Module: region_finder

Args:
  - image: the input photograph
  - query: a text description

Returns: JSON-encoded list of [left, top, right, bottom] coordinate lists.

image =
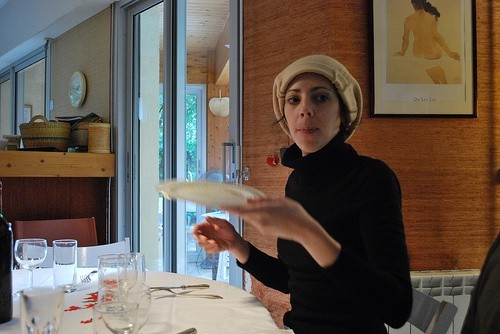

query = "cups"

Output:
[[53, 239, 77, 293], [93, 301, 139, 334], [20, 286, 64, 334], [88, 123, 110, 153]]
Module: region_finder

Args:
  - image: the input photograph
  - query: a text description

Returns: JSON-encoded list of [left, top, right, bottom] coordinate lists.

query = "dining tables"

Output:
[[0, 267, 294, 333]]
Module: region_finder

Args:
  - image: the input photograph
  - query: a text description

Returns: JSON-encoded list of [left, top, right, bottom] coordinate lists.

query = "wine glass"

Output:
[[14, 239, 47, 296], [98, 252, 150, 334]]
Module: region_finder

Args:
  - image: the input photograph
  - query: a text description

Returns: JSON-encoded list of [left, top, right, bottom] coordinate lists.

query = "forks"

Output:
[[80, 271, 98, 282], [152, 288, 223, 300]]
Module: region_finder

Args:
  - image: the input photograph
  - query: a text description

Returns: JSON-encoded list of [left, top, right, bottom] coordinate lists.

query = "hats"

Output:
[[272, 55, 363, 144]]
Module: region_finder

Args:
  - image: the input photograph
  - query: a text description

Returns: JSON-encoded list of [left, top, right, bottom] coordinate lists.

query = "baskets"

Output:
[[19, 115, 71, 152]]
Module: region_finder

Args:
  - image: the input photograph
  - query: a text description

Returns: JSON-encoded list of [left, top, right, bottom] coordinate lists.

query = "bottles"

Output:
[[0, 182, 13, 324]]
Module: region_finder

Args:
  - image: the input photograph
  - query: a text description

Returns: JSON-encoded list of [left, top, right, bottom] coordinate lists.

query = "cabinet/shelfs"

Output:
[[1, 148, 116, 247]]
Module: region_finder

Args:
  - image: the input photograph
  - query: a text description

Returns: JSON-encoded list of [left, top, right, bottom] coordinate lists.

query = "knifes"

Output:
[[149, 284, 209, 289]]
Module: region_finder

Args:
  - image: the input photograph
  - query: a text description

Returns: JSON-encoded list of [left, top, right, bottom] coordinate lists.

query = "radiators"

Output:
[[381, 268, 484, 334]]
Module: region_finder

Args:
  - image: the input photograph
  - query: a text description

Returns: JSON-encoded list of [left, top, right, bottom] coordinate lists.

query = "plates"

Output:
[[158, 181, 267, 207]]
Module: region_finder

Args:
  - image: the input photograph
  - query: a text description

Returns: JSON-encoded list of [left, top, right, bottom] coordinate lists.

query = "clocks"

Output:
[[68, 72, 86, 108]]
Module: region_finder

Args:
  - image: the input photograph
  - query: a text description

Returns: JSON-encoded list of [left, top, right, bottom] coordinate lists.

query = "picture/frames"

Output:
[[366, 0, 479, 119]]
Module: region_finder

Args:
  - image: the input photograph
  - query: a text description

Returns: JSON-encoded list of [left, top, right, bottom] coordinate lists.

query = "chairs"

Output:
[[7, 216, 99, 268], [397, 288, 458, 333], [21, 237, 131, 269]]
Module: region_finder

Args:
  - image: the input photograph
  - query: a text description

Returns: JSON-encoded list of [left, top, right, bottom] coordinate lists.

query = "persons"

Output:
[[191, 54, 413, 334]]
